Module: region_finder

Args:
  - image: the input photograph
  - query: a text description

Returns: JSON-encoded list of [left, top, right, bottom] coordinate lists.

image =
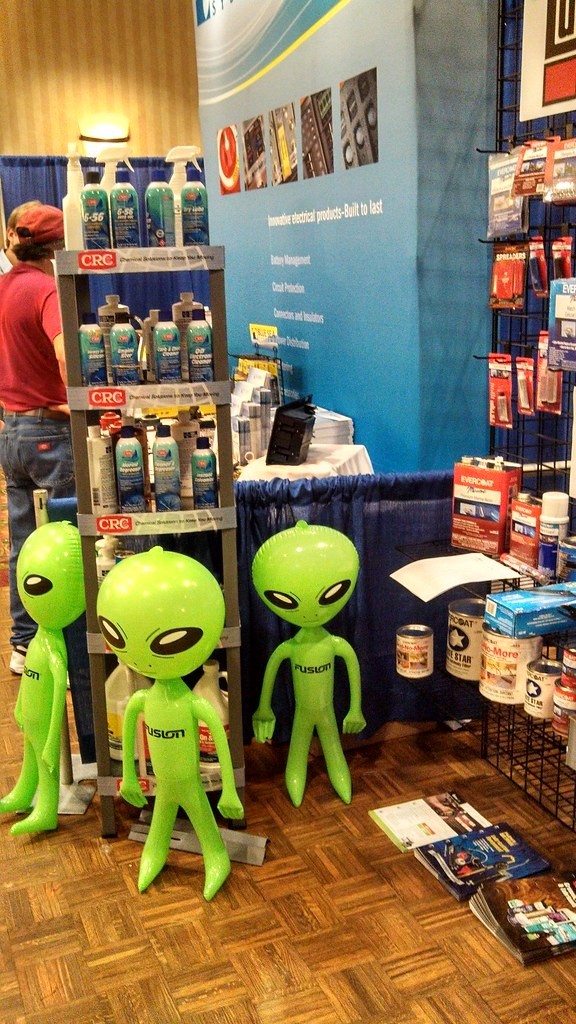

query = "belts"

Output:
[[3, 407, 68, 422]]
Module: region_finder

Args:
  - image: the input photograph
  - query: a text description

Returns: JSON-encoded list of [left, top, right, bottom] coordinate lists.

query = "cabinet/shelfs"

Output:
[[54, 247, 246, 837]]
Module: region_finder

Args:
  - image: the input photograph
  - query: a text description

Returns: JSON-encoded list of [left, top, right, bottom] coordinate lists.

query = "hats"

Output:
[[15, 205, 64, 245]]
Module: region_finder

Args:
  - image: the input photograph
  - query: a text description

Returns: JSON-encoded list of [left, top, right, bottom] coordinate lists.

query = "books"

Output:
[[212, 365, 356, 467], [368, 790, 576, 967]]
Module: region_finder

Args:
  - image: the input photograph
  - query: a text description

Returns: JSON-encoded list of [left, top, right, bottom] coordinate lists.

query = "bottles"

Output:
[[103, 656, 229, 781]]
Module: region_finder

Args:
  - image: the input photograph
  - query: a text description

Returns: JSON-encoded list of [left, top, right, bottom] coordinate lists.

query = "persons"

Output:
[[0, 200, 85, 686]]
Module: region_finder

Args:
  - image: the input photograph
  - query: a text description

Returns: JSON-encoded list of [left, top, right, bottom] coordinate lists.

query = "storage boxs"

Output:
[[484, 581, 576, 640], [448, 461, 520, 556]]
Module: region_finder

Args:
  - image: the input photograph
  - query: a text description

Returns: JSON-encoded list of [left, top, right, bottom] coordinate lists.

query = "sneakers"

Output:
[[10, 642, 27, 674]]
